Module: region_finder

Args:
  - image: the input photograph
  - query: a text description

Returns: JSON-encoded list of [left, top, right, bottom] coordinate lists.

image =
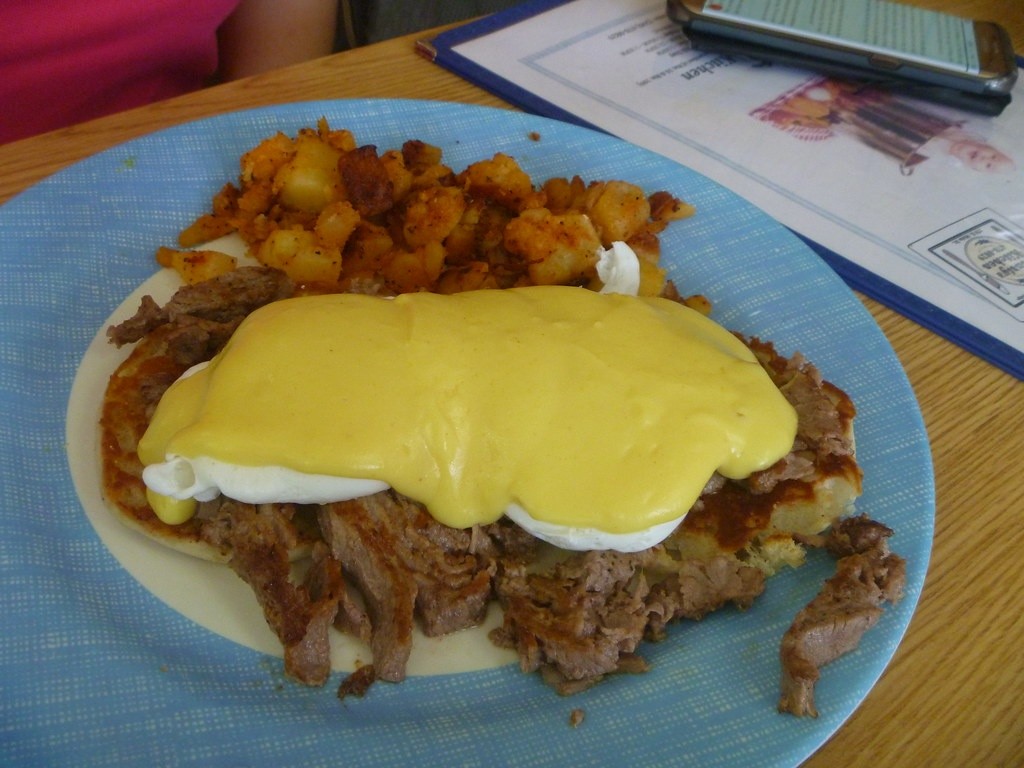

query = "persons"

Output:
[[0, 0, 340, 147]]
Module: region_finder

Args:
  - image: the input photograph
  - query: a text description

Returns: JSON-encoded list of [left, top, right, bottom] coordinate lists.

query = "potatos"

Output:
[[152, 113, 698, 293]]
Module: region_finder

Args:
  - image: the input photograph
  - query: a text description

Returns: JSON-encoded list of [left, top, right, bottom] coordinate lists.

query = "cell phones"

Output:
[[663, 0, 1018, 100]]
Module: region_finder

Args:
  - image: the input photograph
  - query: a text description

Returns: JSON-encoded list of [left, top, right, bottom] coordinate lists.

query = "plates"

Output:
[[0, 98, 935, 768]]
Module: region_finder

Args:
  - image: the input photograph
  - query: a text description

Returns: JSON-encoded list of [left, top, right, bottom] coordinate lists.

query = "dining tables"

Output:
[[0, 0, 1024, 768]]
